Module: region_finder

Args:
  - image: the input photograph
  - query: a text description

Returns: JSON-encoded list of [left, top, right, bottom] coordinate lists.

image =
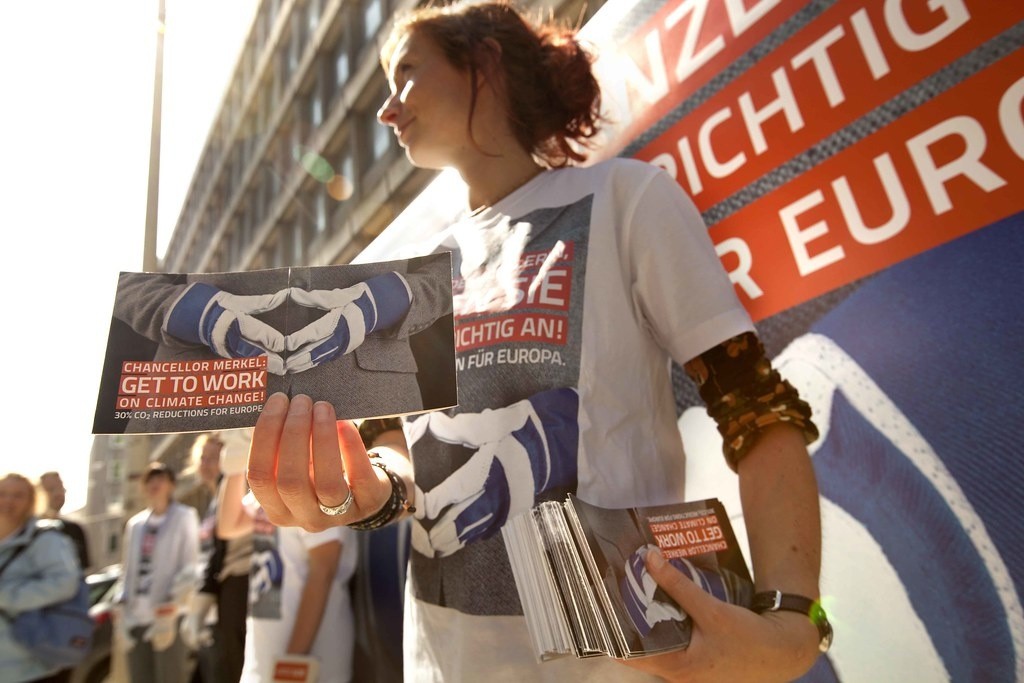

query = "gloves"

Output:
[[422, 387, 581, 559], [162, 282, 291, 377], [619, 544, 730, 639], [286, 271, 413, 376], [399, 412, 437, 560]]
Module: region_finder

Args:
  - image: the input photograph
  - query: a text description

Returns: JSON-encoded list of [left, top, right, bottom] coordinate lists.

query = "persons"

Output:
[[248, 0, 822, 683], [0, 435, 360, 683], [113, 252, 453, 435], [579, 497, 753, 652]]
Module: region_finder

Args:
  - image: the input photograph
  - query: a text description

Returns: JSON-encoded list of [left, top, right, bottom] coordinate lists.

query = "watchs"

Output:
[[754, 590, 834, 653]]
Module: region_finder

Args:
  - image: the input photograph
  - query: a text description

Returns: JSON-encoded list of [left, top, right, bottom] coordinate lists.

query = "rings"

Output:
[[318, 484, 355, 516]]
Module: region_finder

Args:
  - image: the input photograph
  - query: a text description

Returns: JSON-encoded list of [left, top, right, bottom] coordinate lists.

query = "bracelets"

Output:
[[346, 452, 416, 533]]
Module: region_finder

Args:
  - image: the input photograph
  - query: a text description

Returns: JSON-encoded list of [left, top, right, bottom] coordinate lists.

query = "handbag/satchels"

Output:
[[1, 527, 93, 671]]
